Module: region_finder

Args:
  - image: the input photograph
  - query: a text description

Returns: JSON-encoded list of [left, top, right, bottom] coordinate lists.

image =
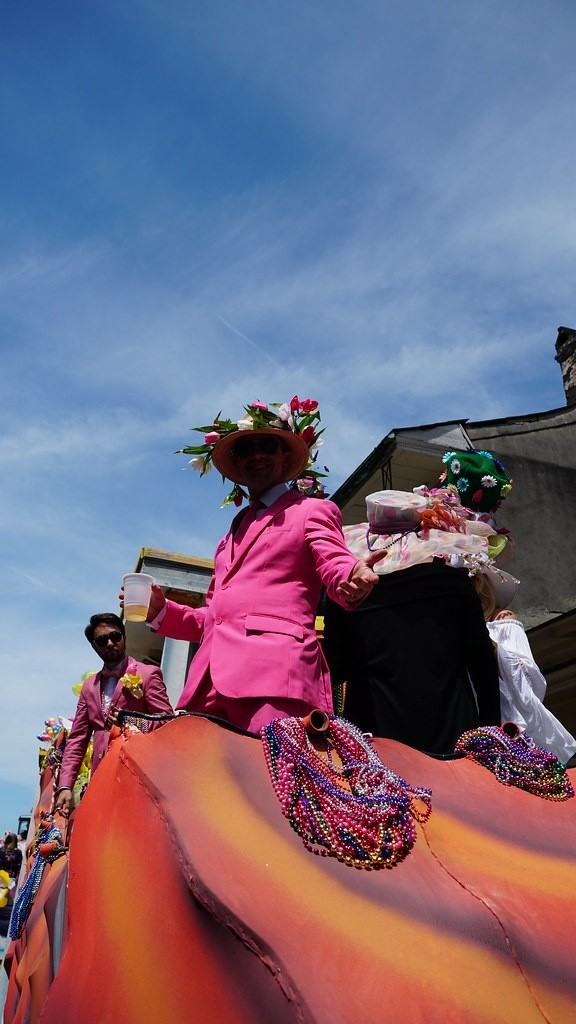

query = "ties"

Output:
[[232, 499, 263, 561]]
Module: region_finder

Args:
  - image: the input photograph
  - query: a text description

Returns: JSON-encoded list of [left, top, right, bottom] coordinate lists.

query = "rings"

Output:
[[56, 806, 61, 810]]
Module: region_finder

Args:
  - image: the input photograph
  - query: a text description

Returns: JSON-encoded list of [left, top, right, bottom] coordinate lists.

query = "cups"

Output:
[[123, 573, 155, 622]]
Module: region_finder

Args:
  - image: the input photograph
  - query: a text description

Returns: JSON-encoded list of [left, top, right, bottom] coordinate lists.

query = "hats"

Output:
[[342, 489, 488, 575], [212, 411, 309, 488]]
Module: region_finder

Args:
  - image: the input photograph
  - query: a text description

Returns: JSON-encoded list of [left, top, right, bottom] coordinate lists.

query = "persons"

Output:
[[471, 570, 576, 768], [0, 833, 23, 878], [54, 611, 172, 819], [119, 422, 385, 736]]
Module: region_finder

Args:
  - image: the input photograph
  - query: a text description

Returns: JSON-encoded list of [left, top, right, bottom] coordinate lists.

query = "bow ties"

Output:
[[101, 668, 122, 678]]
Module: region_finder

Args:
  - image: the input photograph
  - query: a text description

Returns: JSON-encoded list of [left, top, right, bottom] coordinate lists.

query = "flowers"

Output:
[[173, 394, 331, 510], [438, 448, 514, 513]]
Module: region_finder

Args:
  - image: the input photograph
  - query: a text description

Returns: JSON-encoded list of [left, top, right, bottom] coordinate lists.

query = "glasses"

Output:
[[234, 436, 285, 457], [93, 631, 122, 647]]
